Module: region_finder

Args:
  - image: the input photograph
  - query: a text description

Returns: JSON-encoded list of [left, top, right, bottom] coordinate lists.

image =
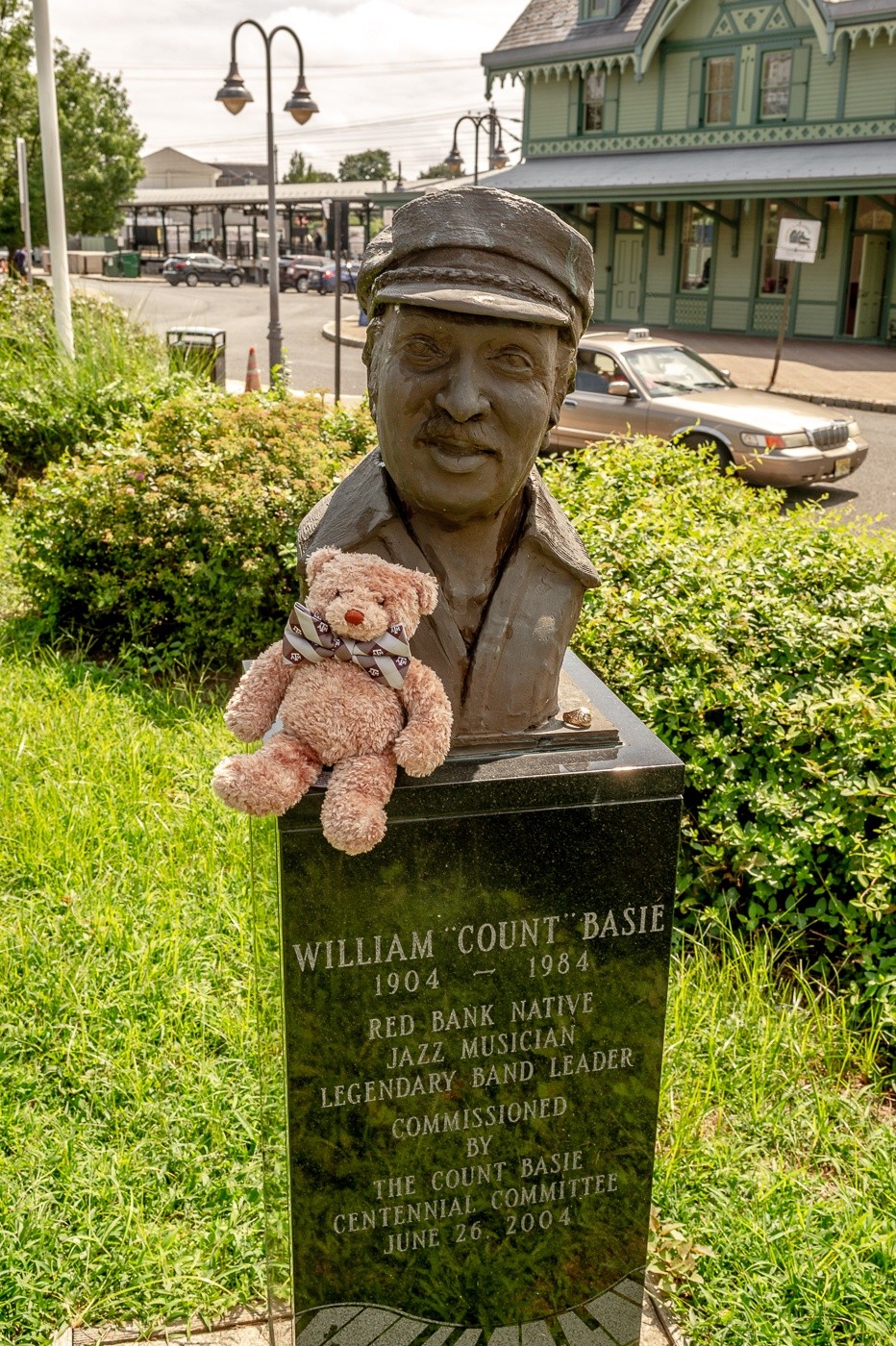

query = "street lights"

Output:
[[212, 18, 320, 390], [444, 115, 512, 187]]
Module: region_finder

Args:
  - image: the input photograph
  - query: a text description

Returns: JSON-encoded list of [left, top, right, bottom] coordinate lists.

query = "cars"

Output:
[[539, 328, 869, 490]]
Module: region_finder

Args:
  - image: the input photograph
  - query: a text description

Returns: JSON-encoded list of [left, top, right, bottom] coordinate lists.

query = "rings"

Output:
[[563, 707, 592, 728]]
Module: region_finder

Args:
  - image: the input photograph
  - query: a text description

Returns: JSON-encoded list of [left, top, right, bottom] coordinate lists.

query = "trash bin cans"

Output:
[[163, 327, 225, 386], [120, 252, 140, 279], [102, 255, 119, 277]]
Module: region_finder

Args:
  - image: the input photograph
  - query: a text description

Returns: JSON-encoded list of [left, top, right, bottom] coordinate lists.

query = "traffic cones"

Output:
[[245, 347, 263, 391]]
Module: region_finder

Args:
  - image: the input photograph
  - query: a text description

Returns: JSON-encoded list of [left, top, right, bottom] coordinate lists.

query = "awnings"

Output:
[[461, 139, 896, 204]]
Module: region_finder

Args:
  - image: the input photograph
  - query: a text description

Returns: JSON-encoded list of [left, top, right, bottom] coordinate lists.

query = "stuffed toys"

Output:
[[210, 548, 449, 854]]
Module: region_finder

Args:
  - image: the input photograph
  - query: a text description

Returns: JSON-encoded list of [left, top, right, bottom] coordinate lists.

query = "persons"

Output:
[[292, 187, 602, 736], [314, 231, 322, 250]]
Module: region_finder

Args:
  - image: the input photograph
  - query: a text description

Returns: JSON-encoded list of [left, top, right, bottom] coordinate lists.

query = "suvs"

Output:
[[162, 253, 247, 287], [265, 254, 364, 296]]
